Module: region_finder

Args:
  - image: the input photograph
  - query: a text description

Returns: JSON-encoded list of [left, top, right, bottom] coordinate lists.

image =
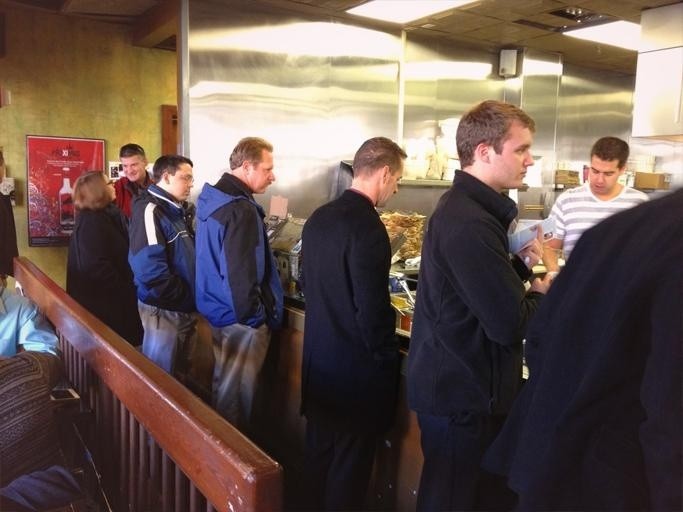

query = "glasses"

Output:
[[170, 171, 194, 184]]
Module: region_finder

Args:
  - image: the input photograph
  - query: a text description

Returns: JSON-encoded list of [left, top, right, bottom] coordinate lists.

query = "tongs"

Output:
[[399, 279, 415, 310]]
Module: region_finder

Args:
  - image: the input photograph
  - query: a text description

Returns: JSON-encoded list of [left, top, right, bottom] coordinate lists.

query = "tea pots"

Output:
[[402, 119, 448, 180]]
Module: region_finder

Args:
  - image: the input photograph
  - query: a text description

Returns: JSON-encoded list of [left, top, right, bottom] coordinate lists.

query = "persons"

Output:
[[112, 143, 152, 221], [66, 170, 144, 348], [1, 349, 61, 489], [506, 178, 683, 512], [539, 136, 651, 283], [128, 154, 198, 377], [0, 272, 60, 353], [298, 137, 408, 511], [195, 136, 284, 450], [406, 99, 553, 512]]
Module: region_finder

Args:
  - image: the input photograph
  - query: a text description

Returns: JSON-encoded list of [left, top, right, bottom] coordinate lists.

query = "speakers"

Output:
[[499, 48, 517, 77]]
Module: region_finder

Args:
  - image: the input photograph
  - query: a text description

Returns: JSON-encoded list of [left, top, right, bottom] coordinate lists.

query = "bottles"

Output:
[[59, 167, 75, 225]]
